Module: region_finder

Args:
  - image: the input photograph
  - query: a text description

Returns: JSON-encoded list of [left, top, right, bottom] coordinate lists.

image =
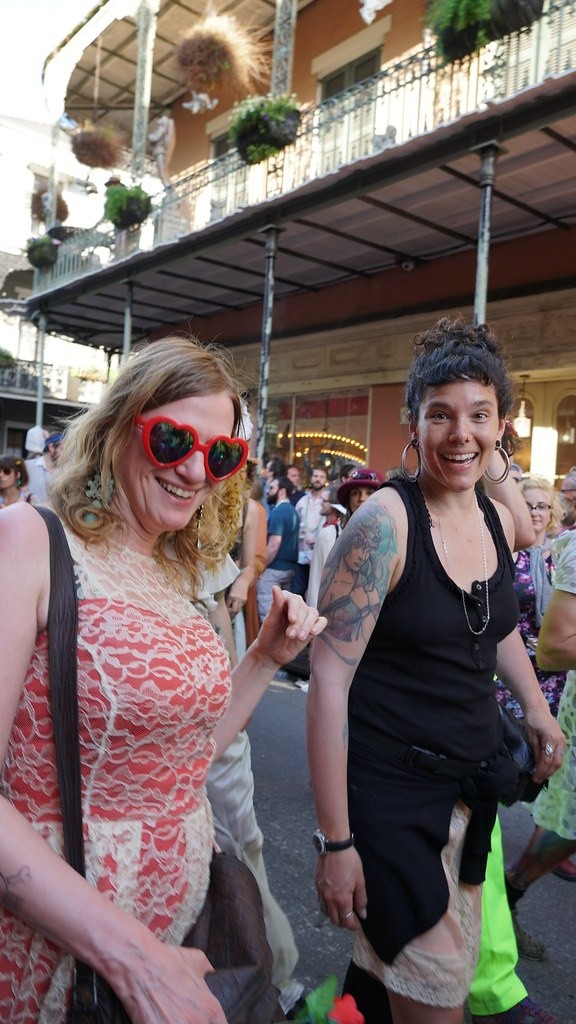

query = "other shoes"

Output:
[[549, 857, 576, 883], [510, 906, 548, 961], [471, 996, 561, 1024]]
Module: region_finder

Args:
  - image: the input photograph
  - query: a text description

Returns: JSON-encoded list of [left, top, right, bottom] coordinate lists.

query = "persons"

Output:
[[182, 91, 219, 115], [78, 175, 98, 196], [95, 173, 120, 228], [0, 432, 576, 1023], [147, 110, 176, 188], [305, 319, 567, 1024], [0, 334, 328, 1023]]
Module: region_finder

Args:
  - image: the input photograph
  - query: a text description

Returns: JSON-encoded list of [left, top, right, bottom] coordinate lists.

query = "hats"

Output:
[[336, 469, 386, 510], [321, 486, 348, 515]]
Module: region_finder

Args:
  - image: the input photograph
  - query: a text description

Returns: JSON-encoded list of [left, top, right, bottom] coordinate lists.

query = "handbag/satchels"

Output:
[[67, 851, 289, 1024]]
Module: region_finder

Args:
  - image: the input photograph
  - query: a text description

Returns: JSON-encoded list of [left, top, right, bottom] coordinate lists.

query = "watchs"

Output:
[[310, 829, 355, 857]]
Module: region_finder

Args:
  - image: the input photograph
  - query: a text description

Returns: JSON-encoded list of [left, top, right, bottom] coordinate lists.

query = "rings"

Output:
[[542, 740, 553, 756], [341, 911, 352, 921]]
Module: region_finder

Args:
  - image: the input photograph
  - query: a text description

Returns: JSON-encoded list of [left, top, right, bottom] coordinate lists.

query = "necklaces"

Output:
[[424, 487, 491, 635]]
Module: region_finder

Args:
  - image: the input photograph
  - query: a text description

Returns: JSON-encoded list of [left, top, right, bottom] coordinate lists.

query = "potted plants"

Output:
[[106, 186, 149, 224], [228, 97, 304, 163], [429, 0, 543, 63], [22, 237, 58, 270]]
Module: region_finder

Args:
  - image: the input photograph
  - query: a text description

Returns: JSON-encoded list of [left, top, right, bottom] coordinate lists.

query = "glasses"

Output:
[[350, 471, 383, 485], [526, 501, 551, 512], [0, 468, 17, 476], [132, 414, 249, 481]]
